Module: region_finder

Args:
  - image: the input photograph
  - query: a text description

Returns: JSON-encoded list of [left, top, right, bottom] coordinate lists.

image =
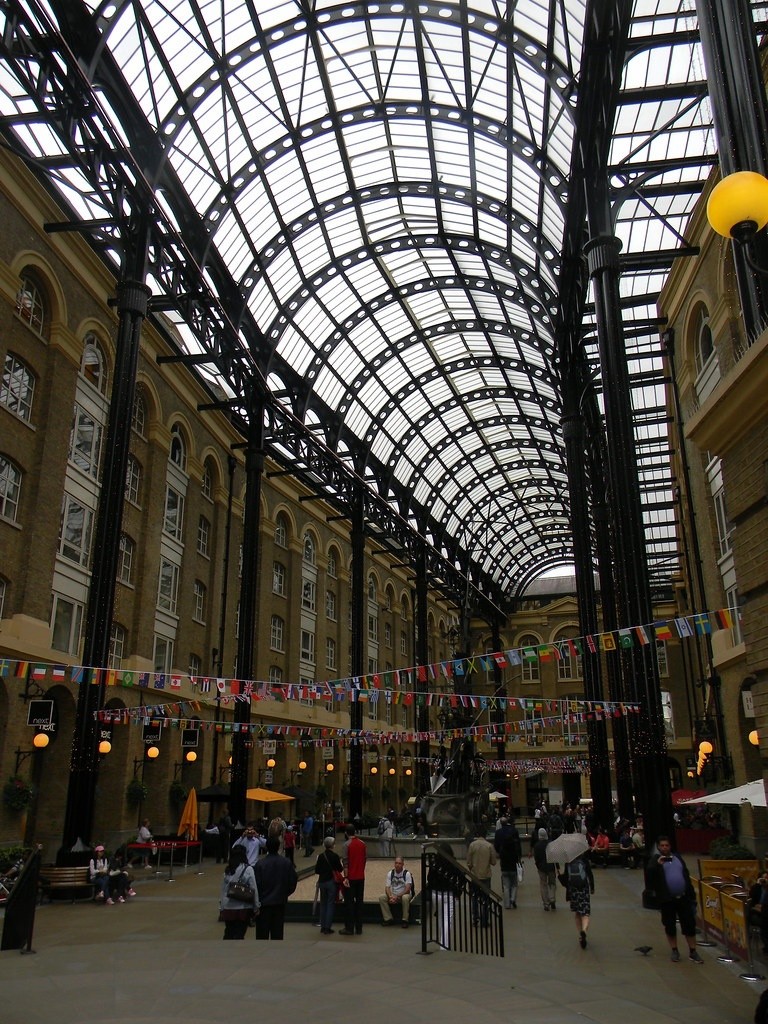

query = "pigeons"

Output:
[[633, 946, 654, 956]]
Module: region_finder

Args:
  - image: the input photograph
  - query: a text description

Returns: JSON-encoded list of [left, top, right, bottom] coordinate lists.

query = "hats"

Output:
[[538, 828, 548, 840], [634, 826, 644, 830], [542, 802, 545, 804], [324, 836, 335, 848], [288, 825, 293, 830], [416, 808, 422, 813], [96, 846, 105, 851]]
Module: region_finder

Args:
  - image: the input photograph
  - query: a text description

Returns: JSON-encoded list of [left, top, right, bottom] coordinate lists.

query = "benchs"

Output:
[[608, 842, 632, 863], [38, 866, 135, 906]]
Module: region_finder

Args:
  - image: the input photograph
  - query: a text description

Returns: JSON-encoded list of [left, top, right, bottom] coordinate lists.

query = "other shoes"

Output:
[[145, 865, 152, 869], [671, 950, 680, 962], [107, 898, 115, 904], [544, 907, 550, 911], [579, 931, 587, 950], [551, 901, 556, 909], [510, 900, 517, 909], [321, 919, 408, 936], [412, 834, 418, 840], [127, 888, 137, 896], [127, 863, 132, 868], [118, 896, 126, 903], [689, 950, 704, 963], [624, 867, 630, 870], [425, 835, 428, 840], [98, 890, 104, 897]]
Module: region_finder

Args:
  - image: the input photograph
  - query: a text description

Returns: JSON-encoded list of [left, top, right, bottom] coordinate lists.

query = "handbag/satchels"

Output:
[[152, 843, 157, 855], [332, 870, 344, 883], [642, 889, 672, 910], [558, 873, 565, 886], [226, 864, 255, 904], [516, 862, 523, 882], [135, 833, 147, 844]]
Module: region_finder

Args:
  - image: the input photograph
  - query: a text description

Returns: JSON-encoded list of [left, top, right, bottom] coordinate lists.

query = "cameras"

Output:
[[663, 856, 672, 862]]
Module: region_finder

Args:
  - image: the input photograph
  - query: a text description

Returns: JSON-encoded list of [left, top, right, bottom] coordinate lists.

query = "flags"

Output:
[[0, 603, 746, 779]]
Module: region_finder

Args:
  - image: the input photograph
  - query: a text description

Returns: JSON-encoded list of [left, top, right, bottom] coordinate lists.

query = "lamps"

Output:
[[748, 728, 759, 753], [87, 740, 112, 775], [400, 769, 412, 783], [705, 170, 768, 275], [173, 750, 199, 779], [365, 767, 379, 785], [686, 740, 735, 783], [291, 761, 307, 782], [14, 732, 48, 776], [259, 758, 276, 782], [219, 757, 232, 779], [318, 763, 339, 780], [383, 767, 396, 783], [133, 746, 160, 778]]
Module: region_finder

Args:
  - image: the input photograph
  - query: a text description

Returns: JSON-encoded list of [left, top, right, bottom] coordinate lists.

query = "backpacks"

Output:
[[86, 857, 105, 884], [391, 869, 415, 901], [377, 820, 388, 835], [564, 856, 587, 887]]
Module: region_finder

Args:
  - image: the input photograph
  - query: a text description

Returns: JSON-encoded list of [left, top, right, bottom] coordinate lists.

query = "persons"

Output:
[[3, 791, 768, 964]]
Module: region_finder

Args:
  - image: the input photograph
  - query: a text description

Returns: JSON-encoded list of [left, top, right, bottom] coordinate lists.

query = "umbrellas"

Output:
[[682, 779, 768, 808], [178, 787, 198, 867], [246, 788, 294, 802]]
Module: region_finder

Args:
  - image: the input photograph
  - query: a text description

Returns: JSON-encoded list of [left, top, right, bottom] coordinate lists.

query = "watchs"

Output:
[[397, 895, 400, 901]]
